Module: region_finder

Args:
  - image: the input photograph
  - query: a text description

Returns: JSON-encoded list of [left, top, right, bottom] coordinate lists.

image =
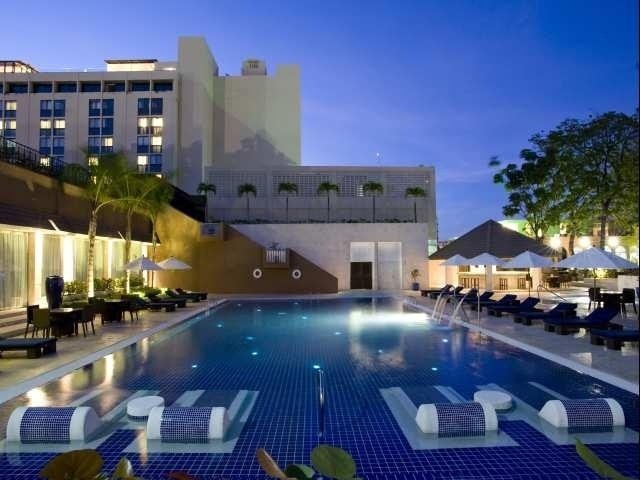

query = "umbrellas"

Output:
[[466, 252, 504, 279], [125, 254, 162, 273], [556, 246, 639, 310], [424, 218, 562, 260], [158, 256, 192, 270], [500, 252, 554, 297], [440, 253, 470, 266]]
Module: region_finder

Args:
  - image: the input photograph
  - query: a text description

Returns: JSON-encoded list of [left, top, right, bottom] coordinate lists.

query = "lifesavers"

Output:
[[253, 269, 262, 279], [292, 268, 302, 279]]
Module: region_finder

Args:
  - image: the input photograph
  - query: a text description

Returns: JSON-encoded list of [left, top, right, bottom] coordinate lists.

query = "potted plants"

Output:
[[411, 269, 420, 291]]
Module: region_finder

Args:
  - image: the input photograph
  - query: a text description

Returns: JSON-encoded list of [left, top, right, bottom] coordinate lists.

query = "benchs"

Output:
[[588, 327, 640, 351], [0, 336, 58, 360]]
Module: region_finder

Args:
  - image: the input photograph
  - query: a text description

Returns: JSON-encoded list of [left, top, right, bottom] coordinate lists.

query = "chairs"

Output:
[[88, 293, 139, 325], [23, 304, 101, 339], [419, 284, 638, 336], [136, 287, 208, 312]]
